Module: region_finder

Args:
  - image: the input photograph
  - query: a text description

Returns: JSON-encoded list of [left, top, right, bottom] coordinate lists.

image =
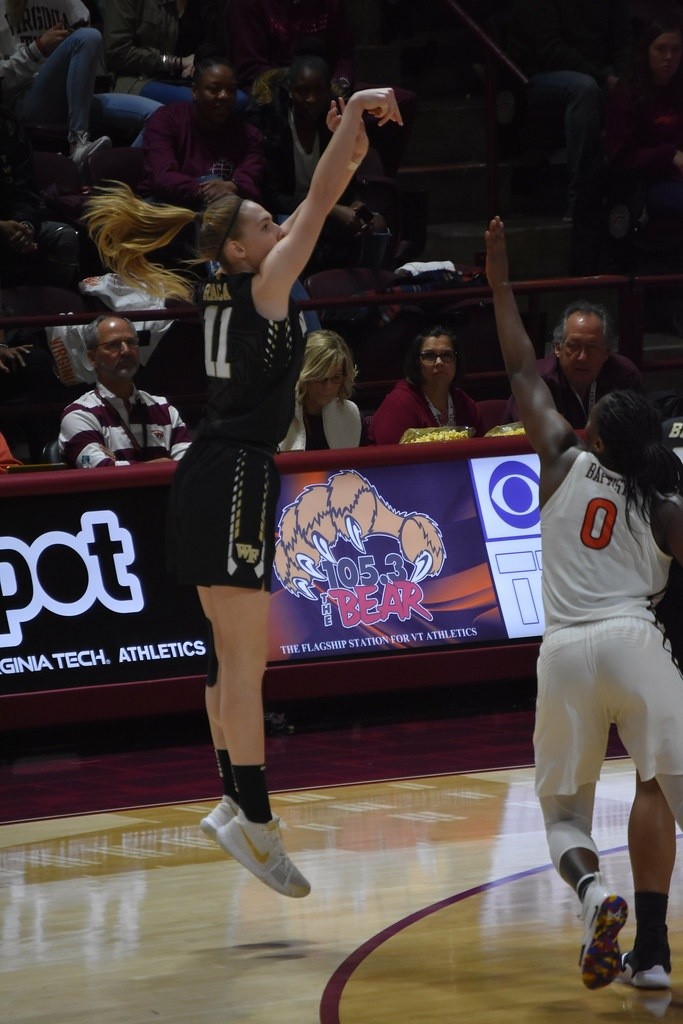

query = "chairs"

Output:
[[0, 0, 682, 433]]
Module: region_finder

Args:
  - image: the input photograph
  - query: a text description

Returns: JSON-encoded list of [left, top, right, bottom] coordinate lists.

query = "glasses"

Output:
[[96, 338, 139, 350], [419, 351, 459, 363], [315, 374, 346, 385]]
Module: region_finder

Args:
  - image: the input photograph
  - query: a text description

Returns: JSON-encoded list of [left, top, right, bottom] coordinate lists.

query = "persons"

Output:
[[485, 215, 683, 990], [0, 0, 683, 444], [81, 83, 403, 898]]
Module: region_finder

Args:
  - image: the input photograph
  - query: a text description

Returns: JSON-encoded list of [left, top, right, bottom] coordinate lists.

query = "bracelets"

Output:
[[0, 344, 8, 348], [350, 159, 359, 172]]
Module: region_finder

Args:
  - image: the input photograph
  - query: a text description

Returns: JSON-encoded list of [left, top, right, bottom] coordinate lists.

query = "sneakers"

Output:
[[200, 794, 240, 841], [615, 950, 671, 990], [578, 872, 628, 990], [215, 809, 310, 897], [71, 135, 112, 177]]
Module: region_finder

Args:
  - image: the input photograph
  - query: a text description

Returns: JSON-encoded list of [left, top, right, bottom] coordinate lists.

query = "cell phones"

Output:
[[356, 205, 374, 223]]
